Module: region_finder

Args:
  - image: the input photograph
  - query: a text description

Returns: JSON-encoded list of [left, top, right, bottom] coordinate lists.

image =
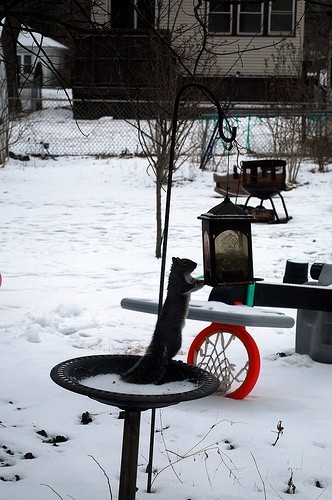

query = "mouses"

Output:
[[119, 256, 204, 384]]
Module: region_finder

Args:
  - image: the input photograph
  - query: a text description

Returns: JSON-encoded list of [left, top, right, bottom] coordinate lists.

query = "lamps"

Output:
[[197, 151, 256, 288]]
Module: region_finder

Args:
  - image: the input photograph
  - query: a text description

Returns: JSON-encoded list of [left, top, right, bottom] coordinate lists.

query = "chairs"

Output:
[[240, 160, 292, 224]]
